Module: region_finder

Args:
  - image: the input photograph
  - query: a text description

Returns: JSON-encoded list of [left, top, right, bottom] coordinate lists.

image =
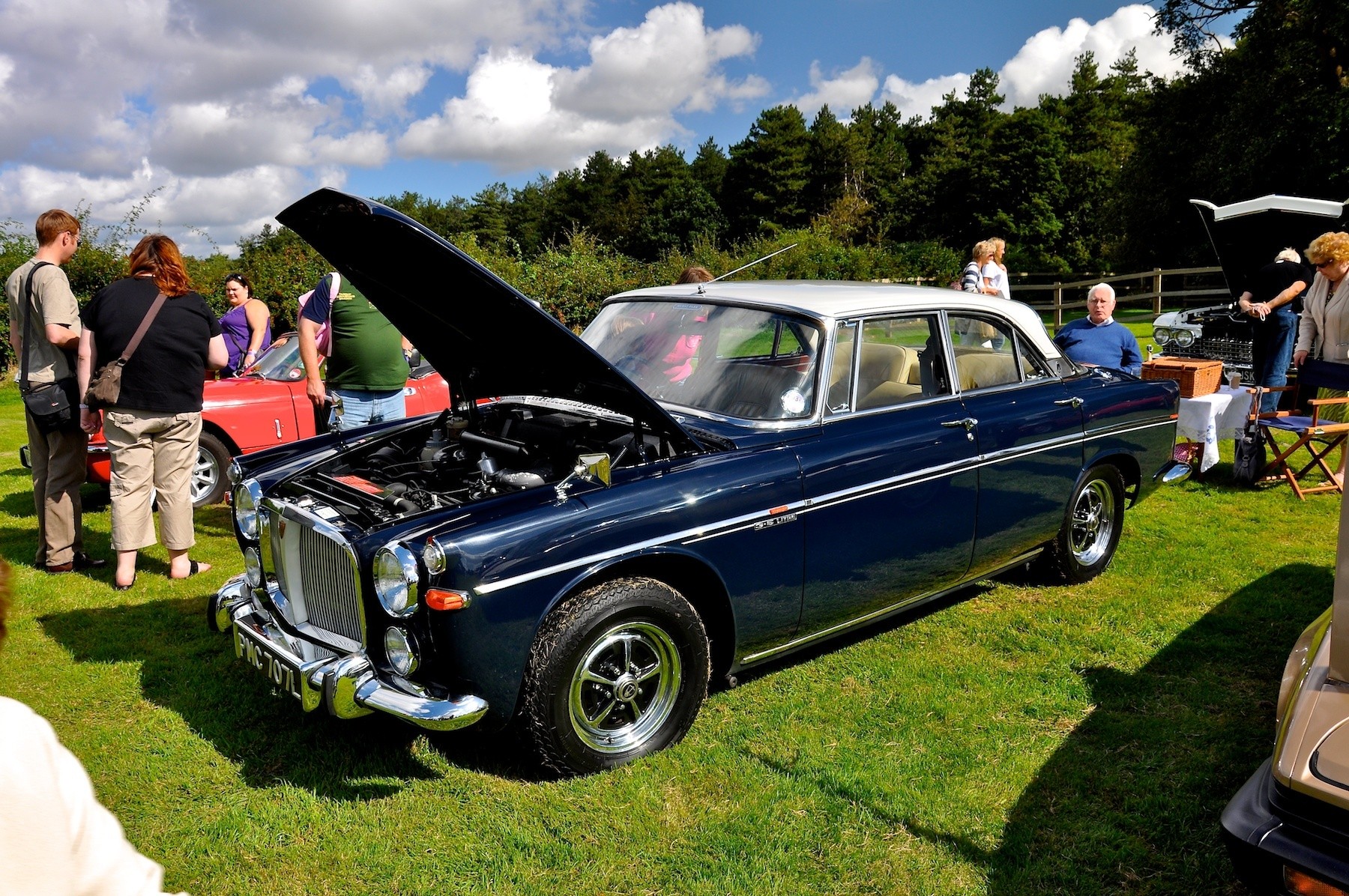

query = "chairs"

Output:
[[682, 362, 813, 419], [1246, 358, 1349, 501]]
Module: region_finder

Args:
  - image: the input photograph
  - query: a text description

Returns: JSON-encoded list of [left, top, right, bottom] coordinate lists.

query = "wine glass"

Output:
[[1224, 364, 1237, 391]]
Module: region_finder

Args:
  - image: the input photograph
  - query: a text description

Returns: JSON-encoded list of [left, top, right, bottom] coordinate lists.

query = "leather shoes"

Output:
[[35, 553, 107, 575]]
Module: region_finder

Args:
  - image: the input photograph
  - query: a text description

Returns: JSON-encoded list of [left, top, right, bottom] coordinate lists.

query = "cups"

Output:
[[1229, 372, 1241, 389]]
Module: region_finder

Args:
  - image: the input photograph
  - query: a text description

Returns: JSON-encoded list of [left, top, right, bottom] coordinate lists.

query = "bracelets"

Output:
[[80, 404, 88, 409], [247, 349, 257, 356]]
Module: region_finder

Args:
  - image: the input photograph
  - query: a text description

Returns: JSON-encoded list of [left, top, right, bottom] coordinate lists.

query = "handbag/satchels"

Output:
[[21, 376, 80, 435], [297, 271, 341, 357], [83, 358, 123, 414], [237, 349, 265, 370], [1232, 431, 1266, 487], [950, 264, 978, 291]]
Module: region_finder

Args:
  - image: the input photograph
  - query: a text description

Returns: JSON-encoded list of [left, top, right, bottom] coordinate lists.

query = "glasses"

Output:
[[1313, 259, 1334, 268], [988, 254, 995, 257]]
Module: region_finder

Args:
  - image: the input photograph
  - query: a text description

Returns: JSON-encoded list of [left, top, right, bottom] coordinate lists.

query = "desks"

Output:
[[1175, 385, 1253, 481]]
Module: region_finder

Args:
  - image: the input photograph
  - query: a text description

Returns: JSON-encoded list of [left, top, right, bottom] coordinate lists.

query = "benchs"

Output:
[[827, 340, 1038, 412]]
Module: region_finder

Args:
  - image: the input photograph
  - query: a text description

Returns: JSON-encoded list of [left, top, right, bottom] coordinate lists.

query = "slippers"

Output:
[[114, 572, 137, 591], [182, 558, 207, 580]]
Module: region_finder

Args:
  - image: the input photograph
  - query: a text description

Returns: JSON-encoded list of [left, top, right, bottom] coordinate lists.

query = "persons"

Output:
[[217, 274, 272, 379], [299, 272, 413, 430], [1050, 283, 1144, 385], [6, 208, 82, 574], [1293, 231, 1349, 487], [608, 264, 716, 397], [1237, 246, 1307, 414], [955, 237, 1011, 352], [78, 231, 229, 590]]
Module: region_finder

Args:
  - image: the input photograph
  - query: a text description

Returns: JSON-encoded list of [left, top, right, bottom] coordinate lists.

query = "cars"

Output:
[[206, 185, 1181, 779], [84, 330, 499, 512], [1152, 194, 1349, 393]]
